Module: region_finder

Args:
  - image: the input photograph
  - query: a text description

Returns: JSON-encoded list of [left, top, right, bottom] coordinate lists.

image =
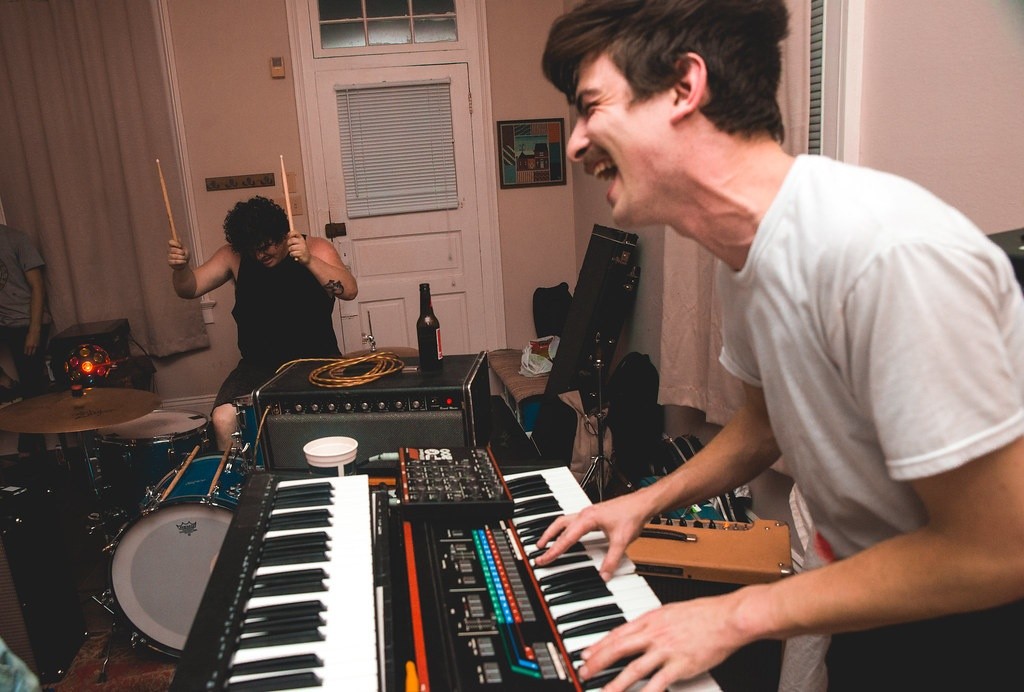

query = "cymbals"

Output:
[[0, 387, 162, 434]]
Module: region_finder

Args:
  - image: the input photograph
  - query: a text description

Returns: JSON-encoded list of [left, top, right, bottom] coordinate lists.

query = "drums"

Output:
[[93, 409, 216, 515], [233, 395, 266, 466], [100, 454, 261, 665]]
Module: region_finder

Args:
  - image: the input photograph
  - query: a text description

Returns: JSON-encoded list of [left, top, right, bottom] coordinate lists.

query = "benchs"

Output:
[[485, 348, 550, 430]]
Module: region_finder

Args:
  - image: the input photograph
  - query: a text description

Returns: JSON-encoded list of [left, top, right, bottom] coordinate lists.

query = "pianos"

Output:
[[166, 459, 723, 692]]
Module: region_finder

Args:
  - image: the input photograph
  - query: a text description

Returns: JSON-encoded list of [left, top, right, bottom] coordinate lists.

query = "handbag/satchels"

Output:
[[518, 335, 561, 378]]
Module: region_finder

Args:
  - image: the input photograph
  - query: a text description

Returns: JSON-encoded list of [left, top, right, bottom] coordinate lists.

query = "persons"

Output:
[[167, 195, 358, 452], [0, 224, 54, 457], [536, 0, 1024, 692]]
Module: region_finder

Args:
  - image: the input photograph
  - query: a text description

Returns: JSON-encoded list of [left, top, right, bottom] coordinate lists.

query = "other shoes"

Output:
[[1, 389, 22, 409]]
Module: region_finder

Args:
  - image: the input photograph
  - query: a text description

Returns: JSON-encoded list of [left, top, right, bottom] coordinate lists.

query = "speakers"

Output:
[[251, 351, 493, 469], [0, 518, 90, 686]]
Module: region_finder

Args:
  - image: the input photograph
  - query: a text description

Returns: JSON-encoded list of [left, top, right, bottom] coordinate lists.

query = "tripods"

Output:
[[578, 333, 637, 504]]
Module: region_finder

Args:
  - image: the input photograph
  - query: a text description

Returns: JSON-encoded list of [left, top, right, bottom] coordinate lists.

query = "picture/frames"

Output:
[[496, 117, 567, 189]]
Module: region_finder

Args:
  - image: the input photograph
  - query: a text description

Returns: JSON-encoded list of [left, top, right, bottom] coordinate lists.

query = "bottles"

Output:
[[415, 283, 443, 372]]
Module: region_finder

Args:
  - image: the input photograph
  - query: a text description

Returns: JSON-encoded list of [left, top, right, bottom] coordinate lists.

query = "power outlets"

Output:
[[287, 197, 303, 216]]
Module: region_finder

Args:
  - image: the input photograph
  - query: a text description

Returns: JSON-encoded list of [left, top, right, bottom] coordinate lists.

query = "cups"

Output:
[[303, 436, 358, 477]]
